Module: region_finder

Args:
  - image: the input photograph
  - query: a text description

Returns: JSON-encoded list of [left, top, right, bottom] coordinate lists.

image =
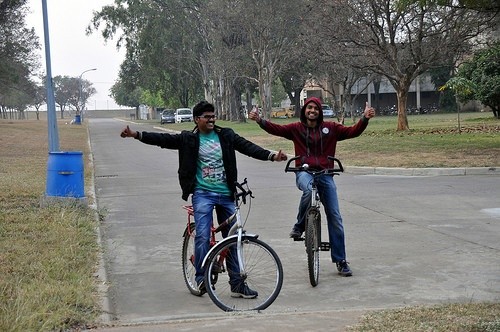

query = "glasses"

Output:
[[198, 115, 217, 120]]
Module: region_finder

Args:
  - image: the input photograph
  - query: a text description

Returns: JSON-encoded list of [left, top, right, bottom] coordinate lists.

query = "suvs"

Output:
[[271, 107, 287, 118], [321, 104, 334, 117], [174, 108, 193, 124], [160, 109, 175, 125]]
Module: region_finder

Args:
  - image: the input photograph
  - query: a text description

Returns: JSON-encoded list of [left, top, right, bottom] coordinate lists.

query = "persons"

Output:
[[120, 101, 288, 299], [248, 96, 375, 276]]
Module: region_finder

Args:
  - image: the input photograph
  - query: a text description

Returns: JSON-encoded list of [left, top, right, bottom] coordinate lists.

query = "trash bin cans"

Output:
[[46, 151, 84, 198], [75, 115, 80, 122]]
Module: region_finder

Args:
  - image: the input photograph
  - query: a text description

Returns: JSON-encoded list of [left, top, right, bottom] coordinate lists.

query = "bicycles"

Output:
[[285, 155, 345, 289], [181, 177, 285, 312]]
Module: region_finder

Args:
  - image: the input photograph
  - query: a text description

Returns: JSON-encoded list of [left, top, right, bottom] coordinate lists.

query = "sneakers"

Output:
[[337, 260, 352, 276], [231, 285, 258, 298], [290, 229, 303, 238], [198, 280, 206, 293]]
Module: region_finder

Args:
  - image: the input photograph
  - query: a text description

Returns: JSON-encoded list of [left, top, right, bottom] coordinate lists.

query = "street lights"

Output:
[[79, 68, 96, 123]]
